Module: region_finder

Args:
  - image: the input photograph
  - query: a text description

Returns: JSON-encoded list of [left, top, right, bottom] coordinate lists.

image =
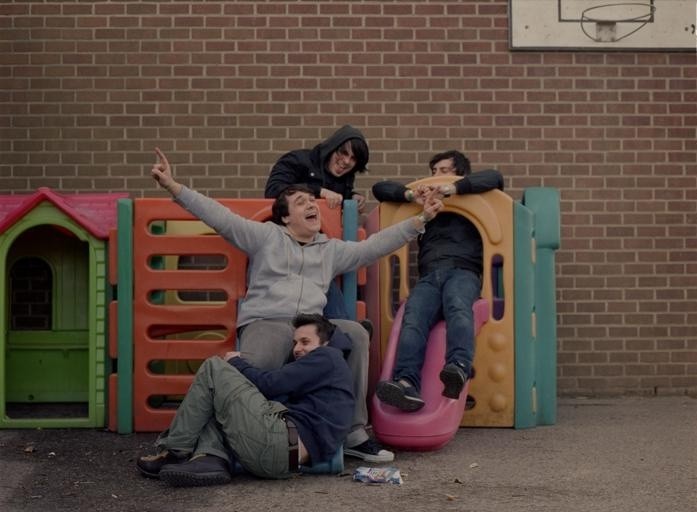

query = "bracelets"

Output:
[[443, 184, 450, 196], [416, 213, 433, 227], [406, 189, 416, 204]]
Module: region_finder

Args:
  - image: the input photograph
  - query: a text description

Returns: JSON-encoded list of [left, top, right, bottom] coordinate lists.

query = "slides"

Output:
[[371, 296, 489, 451]]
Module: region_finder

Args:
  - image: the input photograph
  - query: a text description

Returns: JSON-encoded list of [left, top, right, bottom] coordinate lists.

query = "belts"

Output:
[[286, 420, 298, 472]]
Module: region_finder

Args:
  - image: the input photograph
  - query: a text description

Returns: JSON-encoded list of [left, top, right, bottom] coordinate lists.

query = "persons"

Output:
[[134, 312, 356, 485], [371, 149, 503, 413], [262, 125, 372, 339], [146, 144, 445, 474]]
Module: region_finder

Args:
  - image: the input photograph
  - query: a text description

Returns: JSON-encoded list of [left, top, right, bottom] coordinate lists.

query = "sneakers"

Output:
[[136, 449, 191, 477], [376, 377, 424, 412], [345, 435, 394, 462], [159, 455, 232, 487], [440, 362, 467, 398]]
